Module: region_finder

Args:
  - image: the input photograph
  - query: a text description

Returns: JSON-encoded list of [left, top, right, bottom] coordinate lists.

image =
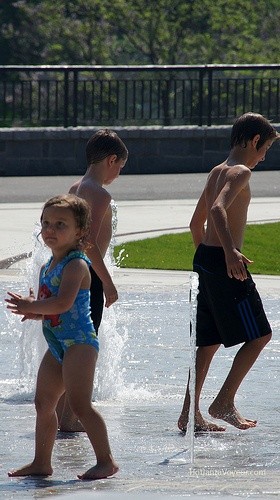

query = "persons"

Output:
[[177, 112, 280, 434], [4, 193, 119, 480], [55, 127, 129, 434]]
